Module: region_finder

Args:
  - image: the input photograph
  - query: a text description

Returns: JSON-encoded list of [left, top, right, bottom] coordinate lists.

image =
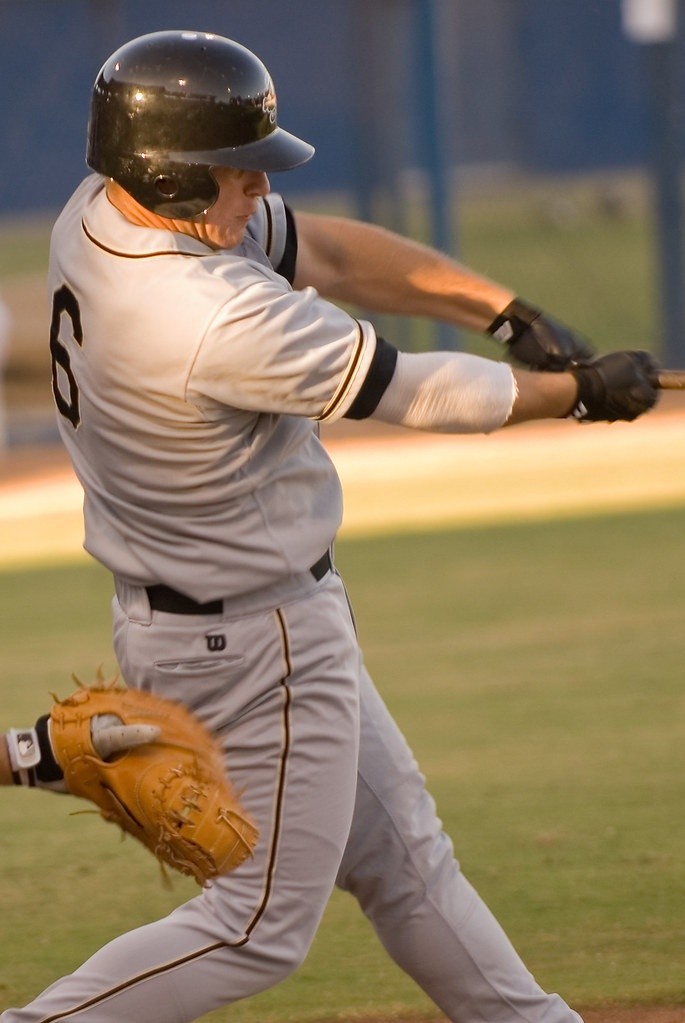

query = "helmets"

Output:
[[86, 31, 316, 219]]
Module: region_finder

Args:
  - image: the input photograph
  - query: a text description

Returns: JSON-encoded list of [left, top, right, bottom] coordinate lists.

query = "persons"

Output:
[[0, 663, 261, 890], [0, 31, 659, 1023]]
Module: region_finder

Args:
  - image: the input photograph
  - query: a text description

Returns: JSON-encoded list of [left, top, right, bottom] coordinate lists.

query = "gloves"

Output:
[[560, 350, 662, 423], [485, 296, 595, 372]]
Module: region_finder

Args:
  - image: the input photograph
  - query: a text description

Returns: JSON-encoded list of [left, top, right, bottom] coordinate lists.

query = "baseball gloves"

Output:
[[50, 682, 260, 890]]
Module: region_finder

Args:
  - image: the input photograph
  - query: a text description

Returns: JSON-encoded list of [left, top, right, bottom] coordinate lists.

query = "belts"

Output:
[[145, 547, 330, 615]]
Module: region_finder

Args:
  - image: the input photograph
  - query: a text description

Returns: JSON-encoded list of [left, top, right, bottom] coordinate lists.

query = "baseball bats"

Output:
[[528, 364, 685, 391]]
